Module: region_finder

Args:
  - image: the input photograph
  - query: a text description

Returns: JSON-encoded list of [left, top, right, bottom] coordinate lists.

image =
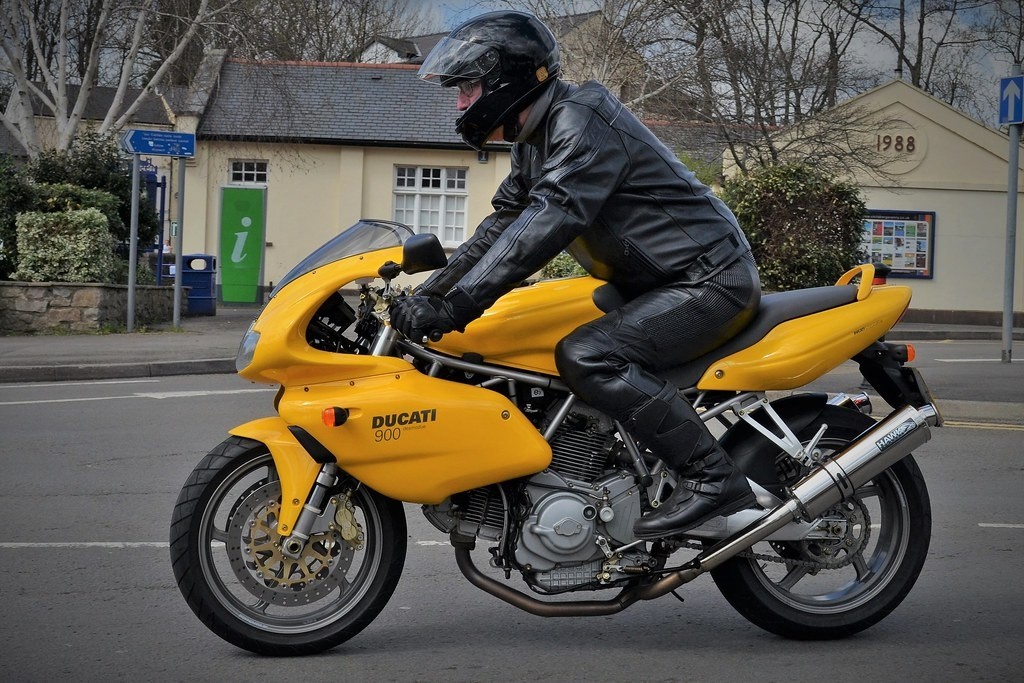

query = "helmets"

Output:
[[439, 10, 560, 152]]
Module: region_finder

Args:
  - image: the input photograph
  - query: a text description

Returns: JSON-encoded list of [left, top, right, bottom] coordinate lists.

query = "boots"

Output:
[[619, 379, 757, 540]]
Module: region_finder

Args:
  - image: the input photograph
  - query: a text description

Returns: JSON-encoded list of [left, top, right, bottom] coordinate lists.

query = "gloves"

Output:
[[388, 283, 484, 344]]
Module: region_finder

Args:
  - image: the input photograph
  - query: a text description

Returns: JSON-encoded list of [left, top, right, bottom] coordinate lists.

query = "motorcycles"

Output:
[[168, 220, 946, 660]]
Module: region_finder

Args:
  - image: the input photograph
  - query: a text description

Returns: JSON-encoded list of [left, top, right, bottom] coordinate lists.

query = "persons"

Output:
[[389, 9, 761, 540]]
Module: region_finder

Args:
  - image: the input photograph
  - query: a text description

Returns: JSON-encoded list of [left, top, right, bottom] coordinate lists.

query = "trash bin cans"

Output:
[[181, 253, 217, 316]]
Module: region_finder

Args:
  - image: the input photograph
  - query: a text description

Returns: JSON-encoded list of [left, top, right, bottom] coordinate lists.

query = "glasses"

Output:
[[456, 78, 480, 97]]
